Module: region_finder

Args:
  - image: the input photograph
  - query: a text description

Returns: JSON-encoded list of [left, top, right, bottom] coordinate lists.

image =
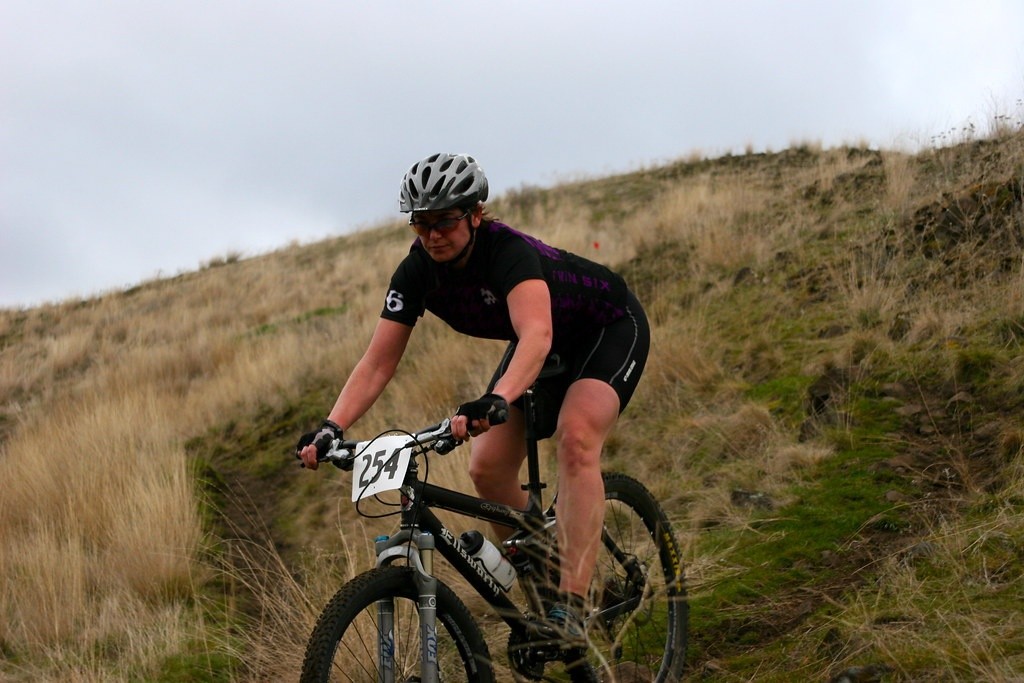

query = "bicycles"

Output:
[[294, 396, 690, 683]]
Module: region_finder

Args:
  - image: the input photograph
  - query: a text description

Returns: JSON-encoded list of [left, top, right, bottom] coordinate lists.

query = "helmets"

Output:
[[399, 152, 489, 214]]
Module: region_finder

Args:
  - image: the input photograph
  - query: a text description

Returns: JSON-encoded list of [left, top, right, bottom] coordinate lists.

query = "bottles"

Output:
[[460, 529, 517, 591]]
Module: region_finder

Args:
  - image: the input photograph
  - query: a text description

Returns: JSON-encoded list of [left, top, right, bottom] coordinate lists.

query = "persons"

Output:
[[296, 154, 650, 650]]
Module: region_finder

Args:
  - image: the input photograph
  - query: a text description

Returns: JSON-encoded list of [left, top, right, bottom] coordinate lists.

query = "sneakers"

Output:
[[525, 599, 588, 660]]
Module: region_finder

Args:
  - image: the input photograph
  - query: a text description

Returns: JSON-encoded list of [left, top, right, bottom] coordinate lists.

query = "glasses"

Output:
[[408, 201, 480, 235]]
